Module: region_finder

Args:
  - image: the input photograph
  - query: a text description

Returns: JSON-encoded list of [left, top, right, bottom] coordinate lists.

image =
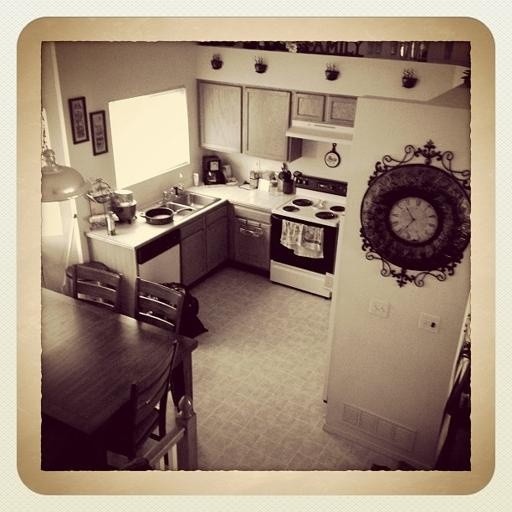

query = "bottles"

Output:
[[277, 163, 286, 192], [283, 171, 293, 193], [107, 211, 116, 236]]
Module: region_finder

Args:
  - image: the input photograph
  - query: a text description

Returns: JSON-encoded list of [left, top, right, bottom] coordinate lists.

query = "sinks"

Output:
[[172, 194, 214, 209], [160, 203, 194, 218]]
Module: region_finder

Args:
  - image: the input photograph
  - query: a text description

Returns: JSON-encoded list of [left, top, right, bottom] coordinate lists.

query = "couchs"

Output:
[[323, 91, 356, 129]]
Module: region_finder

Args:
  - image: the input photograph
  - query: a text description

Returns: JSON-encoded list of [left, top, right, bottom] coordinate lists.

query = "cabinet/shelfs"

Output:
[[180, 215, 207, 287], [195, 79, 243, 153], [227, 205, 270, 272], [290, 90, 326, 125], [242, 85, 304, 163], [206, 203, 233, 273]]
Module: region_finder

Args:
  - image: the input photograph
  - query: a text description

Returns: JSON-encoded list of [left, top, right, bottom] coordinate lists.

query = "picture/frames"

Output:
[[88, 109, 110, 157], [66, 95, 90, 145]]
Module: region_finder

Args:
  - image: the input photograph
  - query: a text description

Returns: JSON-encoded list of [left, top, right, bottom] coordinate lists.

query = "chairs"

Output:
[[134, 275, 187, 409], [72, 263, 124, 313], [105, 339, 181, 472]]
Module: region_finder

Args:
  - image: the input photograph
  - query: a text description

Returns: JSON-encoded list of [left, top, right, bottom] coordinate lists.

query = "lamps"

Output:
[[40, 107, 88, 203]]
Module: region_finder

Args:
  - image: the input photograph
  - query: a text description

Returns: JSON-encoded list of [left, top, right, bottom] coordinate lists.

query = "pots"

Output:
[[140, 207, 193, 226], [324, 143, 341, 168]]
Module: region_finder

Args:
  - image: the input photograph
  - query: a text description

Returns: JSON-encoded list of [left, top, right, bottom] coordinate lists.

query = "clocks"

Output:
[[359, 138, 472, 289]]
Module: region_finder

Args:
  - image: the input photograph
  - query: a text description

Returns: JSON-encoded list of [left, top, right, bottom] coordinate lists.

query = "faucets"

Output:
[[163, 187, 175, 204], [174, 184, 185, 198]]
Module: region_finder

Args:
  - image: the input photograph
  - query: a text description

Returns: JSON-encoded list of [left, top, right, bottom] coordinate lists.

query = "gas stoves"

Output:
[[271, 174, 348, 228]]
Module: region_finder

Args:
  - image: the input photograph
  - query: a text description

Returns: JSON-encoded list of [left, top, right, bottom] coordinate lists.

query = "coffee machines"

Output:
[[202, 155, 223, 185]]
